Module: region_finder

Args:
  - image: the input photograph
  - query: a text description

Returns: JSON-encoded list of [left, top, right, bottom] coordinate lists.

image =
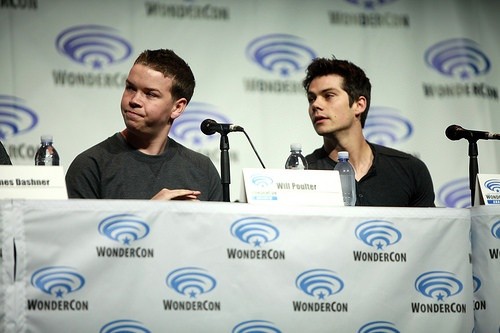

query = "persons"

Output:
[[303, 54, 436, 207], [63, 47, 223, 201]]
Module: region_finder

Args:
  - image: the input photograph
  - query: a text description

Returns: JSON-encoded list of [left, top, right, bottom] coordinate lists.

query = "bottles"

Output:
[[335, 150, 358, 206], [285, 143, 307, 170], [34, 136, 60, 166]]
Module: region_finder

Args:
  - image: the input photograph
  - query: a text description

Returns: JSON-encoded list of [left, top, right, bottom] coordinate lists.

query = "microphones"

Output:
[[445, 124, 500, 140], [201, 119, 244, 135]]
